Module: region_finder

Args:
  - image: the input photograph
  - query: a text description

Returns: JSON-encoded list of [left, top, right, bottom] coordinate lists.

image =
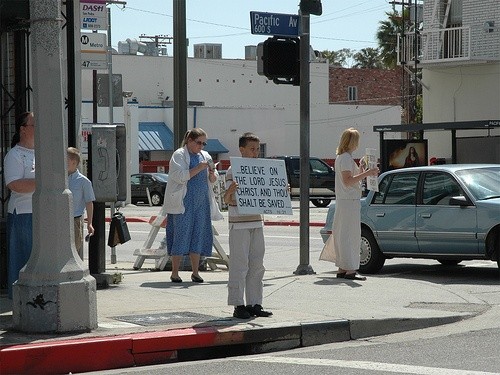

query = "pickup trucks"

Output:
[[265, 156, 336, 207]]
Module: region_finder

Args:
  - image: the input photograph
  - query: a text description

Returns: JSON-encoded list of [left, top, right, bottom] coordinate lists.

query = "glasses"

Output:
[[194, 139, 207, 146]]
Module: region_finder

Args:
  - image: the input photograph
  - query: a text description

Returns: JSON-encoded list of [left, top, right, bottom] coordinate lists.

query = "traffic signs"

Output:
[[250, 11, 299, 37]]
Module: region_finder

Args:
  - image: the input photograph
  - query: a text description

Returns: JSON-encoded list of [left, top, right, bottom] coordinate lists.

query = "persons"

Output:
[[430, 157, 438, 166], [404, 146, 419, 167], [65, 145, 95, 255], [223, 134, 291, 319], [165, 127, 219, 283], [3, 110, 36, 299], [334, 127, 381, 280]]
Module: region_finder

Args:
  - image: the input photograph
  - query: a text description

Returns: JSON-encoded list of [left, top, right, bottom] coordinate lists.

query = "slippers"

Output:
[[343, 272, 367, 280], [337, 272, 346, 278]]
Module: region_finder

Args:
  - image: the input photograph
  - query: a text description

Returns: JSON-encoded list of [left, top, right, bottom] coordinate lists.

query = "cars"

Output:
[[131, 173, 169, 206], [321, 164, 500, 271]]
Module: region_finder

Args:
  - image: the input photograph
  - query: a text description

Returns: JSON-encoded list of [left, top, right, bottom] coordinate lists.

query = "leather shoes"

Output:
[[191, 274, 204, 283], [170, 275, 182, 282], [233, 305, 250, 319], [246, 304, 273, 317]]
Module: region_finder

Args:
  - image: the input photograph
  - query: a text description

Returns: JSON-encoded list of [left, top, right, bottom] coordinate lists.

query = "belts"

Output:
[[74, 215, 82, 218]]
